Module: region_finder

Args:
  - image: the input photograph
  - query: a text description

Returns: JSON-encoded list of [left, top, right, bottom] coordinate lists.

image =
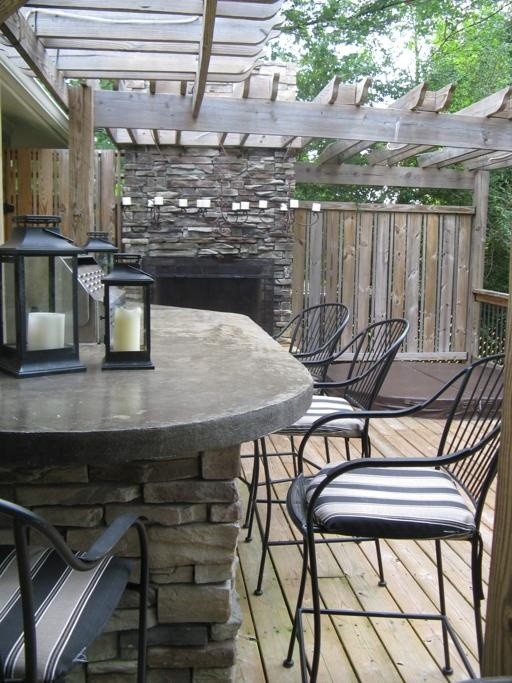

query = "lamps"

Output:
[[121, 174, 321, 229]]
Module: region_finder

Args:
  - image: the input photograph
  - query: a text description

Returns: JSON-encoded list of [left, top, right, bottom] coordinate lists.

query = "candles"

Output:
[[24, 309, 69, 353], [114, 305, 144, 350]]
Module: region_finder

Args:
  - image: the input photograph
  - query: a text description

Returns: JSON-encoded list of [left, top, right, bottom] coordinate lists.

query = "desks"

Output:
[[0, 300, 317, 681]]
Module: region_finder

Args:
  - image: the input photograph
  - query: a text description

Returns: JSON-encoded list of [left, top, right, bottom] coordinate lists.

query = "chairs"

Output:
[[1, 496, 156, 681], [278, 351, 509, 683], [242, 313, 413, 598], [237, 301, 352, 532]]
